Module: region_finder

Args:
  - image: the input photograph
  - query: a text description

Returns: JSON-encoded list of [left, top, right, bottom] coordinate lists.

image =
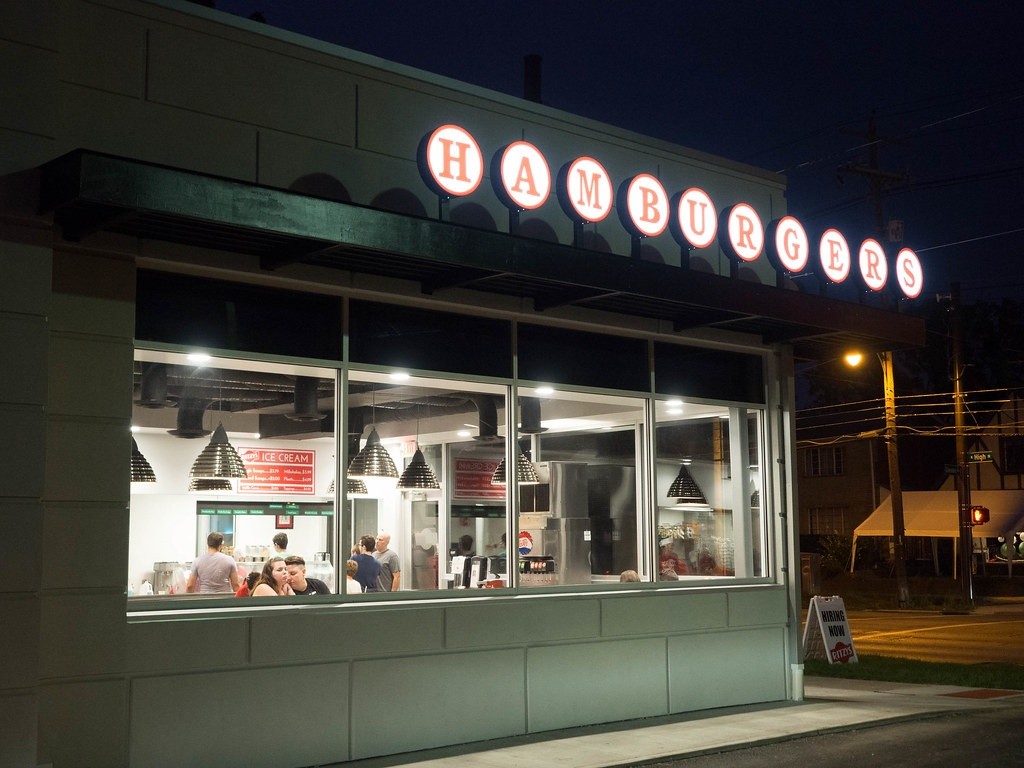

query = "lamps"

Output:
[[752, 490, 760, 506], [131, 436, 157, 482], [328, 471, 367, 495], [188, 400, 232, 491], [345, 383, 400, 478], [396, 404, 440, 490], [491, 439, 541, 485], [668, 465, 709, 507], [188, 370, 248, 478]]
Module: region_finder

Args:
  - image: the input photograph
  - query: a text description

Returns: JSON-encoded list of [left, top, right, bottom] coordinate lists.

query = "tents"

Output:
[[848, 485, 1024, 579]]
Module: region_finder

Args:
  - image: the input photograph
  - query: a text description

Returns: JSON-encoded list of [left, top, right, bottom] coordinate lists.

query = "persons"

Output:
[[236, 572, 261, 597], [346, 559, 363, 595], [497, 532, 506, 557], [269, 532, 292, 559], [283, 555, 332, 595], [620, 569, 641, 582], [458, 535, 476, 557], [252, 557, 296, 596], [186, 531, 239, 595], [350, 535, 381, 594], [371, 532, 401, 592]]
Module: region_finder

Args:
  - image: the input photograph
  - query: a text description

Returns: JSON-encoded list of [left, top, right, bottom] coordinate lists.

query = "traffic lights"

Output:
[[972, 509, 990, 523]]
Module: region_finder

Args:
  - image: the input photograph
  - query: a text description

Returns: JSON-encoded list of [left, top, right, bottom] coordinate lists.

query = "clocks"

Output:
[[276, 515, 293, 528]]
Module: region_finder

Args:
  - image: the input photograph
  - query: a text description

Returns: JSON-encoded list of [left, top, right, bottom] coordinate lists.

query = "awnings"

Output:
[[1, 147, 926, 364]]
